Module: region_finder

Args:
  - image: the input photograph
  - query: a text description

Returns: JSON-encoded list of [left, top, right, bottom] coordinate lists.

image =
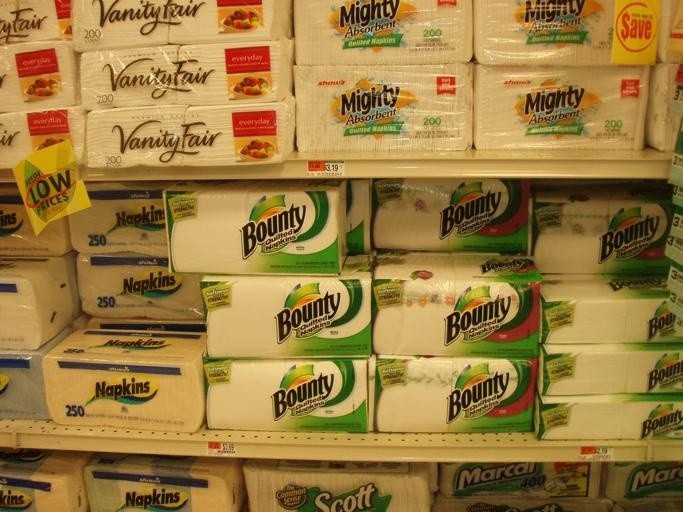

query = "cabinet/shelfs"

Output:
[[0, 150, 683, 464]]
